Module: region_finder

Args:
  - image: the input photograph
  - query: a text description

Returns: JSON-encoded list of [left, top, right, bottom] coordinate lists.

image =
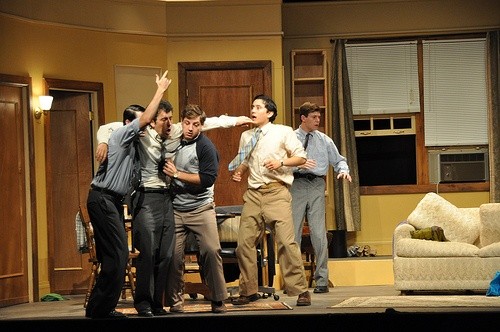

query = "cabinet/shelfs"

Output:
[[290, 48, 328, 138]]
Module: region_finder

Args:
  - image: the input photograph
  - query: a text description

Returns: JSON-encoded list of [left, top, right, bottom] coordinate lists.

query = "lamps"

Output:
[[34, 95, 53, 125]]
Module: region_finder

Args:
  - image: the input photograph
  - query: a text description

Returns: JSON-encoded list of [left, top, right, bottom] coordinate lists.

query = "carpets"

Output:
[[327, 296, 500, 307], [115, 301, 293, 316]]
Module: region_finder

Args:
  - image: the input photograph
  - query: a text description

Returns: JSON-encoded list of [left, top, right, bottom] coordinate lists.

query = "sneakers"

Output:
[[233, 293, 259, 305], [297, 291, 311, 306]]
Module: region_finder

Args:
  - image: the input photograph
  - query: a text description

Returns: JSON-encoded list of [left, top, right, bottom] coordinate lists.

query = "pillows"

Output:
[[407, 192, 479, 244], [479, 203, 500, 247]]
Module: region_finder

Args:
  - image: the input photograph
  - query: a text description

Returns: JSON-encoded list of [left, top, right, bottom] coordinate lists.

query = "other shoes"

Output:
[[138, 307, 154, 317], [152, 309, 167, 316], [211, 300, 227, 314], [92, 310, 128, 319], [86, 312, 92, 317], [314, 286, 329, 293], [169, 300, 185, 313]]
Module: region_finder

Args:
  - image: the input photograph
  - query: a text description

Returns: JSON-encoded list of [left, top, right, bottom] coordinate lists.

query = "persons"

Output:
[[162, 103, 229, 313], [284, 101, 352, 294], [229, 95, 311, 306], [96, 100, 255, 317], [86, 71, 171, 317]]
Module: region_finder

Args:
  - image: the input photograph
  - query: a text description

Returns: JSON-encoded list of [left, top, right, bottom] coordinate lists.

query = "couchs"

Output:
[[393, 192, 500, 296], [183, 205, 274, 299]]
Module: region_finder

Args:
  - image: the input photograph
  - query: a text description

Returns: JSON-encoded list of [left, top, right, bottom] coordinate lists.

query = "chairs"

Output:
[[78, 206, 138, 308]]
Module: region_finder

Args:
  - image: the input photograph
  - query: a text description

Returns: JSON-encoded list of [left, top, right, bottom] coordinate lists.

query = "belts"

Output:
[[91, 183, 125, 202], [141, 188, 169, 193], [293, 172, 316, 181], [258, 181, 285, 189]]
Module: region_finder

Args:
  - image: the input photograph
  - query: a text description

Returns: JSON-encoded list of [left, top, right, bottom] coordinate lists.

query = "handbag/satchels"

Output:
[[411, 226, 446, 242]]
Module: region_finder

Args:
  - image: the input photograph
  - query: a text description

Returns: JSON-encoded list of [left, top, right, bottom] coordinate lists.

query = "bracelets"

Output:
[[280, 159, 284, 166]]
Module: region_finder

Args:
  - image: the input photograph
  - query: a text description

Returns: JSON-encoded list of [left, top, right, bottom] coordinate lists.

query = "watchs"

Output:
[[173, 170, 178, 179]]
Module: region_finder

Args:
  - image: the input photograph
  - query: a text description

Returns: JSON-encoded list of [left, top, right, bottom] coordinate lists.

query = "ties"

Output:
[[158, 137, 167, 179], [134, 134, 141, 188], [304, 133, 310, 150], [229, 129, 262, 170]]
